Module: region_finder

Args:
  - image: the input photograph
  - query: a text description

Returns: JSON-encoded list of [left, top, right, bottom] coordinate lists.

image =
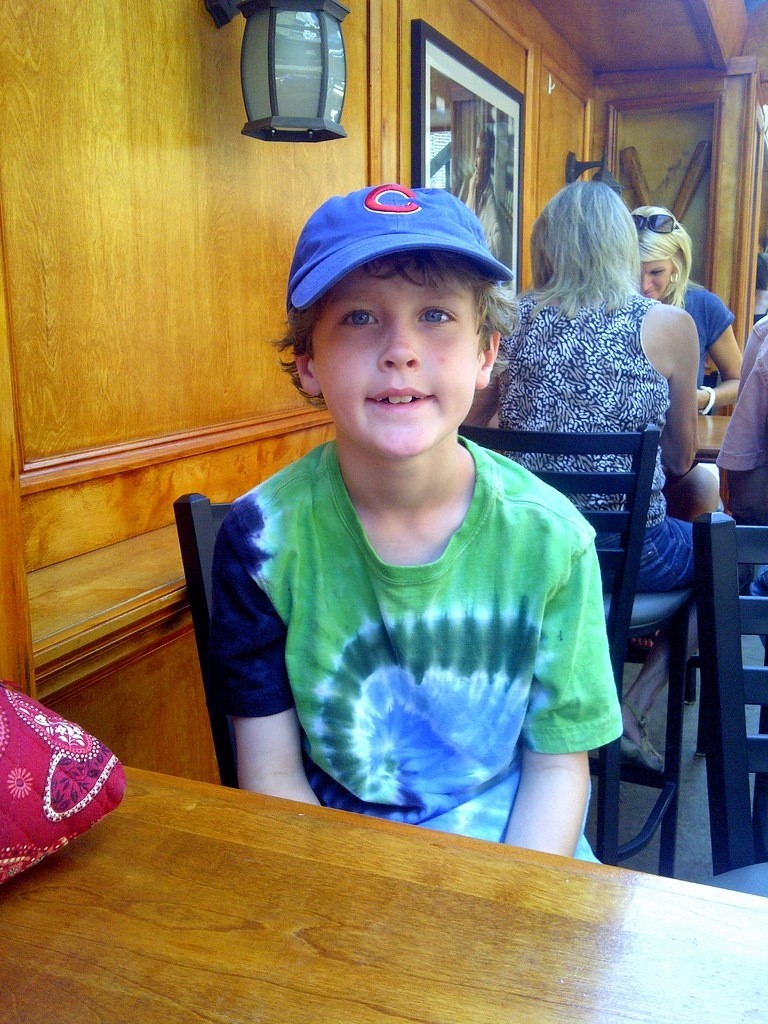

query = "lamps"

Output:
[[204, 0, 352, 146]]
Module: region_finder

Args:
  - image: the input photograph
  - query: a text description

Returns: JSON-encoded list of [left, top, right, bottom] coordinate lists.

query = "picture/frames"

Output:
[[412, 18, 525, 300]]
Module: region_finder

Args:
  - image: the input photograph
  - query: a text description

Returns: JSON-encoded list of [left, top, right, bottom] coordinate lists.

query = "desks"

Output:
[[663, 414, 736, 517], [0, 766, 768, 1024]]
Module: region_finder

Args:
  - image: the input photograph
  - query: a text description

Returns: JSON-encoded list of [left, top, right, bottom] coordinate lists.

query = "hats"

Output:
[[286, 183, 514, 313]]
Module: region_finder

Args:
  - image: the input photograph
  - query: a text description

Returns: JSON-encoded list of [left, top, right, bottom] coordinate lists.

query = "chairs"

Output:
[[167, 434, 768, 878]]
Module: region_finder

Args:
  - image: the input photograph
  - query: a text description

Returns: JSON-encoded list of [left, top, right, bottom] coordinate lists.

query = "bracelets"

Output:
[[698, 386, 715, 415]]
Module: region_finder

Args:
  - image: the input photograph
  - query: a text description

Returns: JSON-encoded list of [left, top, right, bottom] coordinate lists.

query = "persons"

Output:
[[213, 185, 623, 865], [717, 313, 768, 648], [459, 128, 503, 260], [465, 181, 698, 773], [631, 205, 743, 521]]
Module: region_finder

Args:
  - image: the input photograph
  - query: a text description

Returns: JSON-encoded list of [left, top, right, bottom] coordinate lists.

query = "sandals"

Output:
[[615, 699, 666, 772]]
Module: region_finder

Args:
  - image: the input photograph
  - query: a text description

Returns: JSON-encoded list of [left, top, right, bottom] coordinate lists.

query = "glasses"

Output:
[[630, 213, 678, 234]]
